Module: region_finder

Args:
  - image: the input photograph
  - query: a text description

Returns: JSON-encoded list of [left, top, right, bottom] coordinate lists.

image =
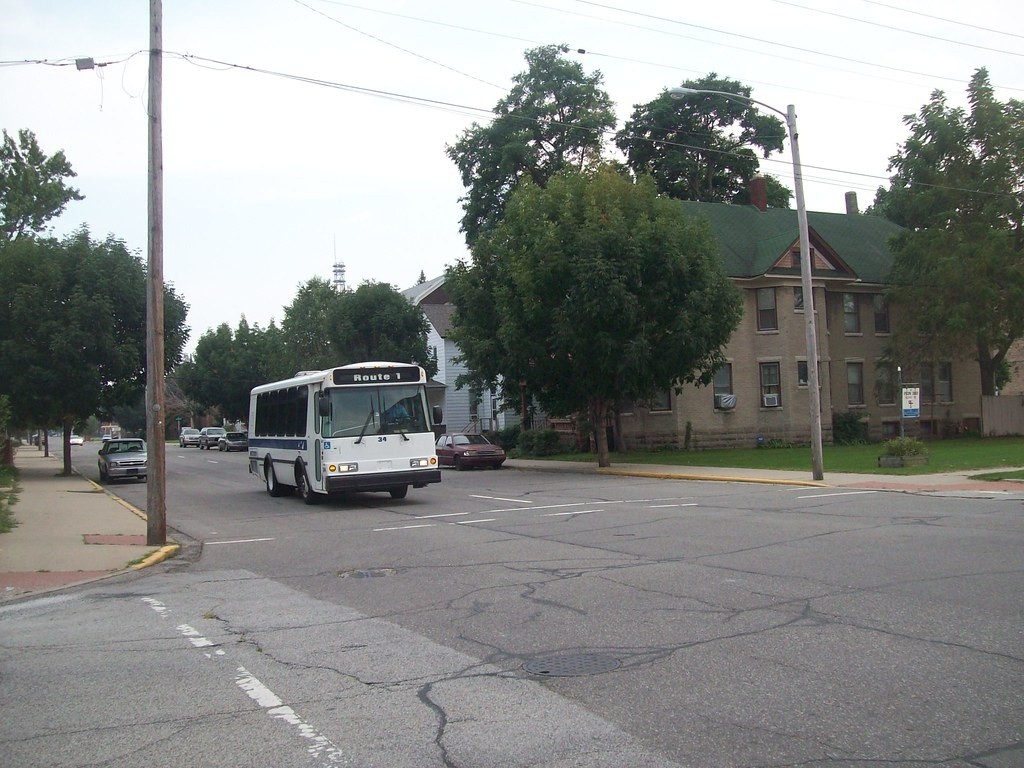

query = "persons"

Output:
[[388, 401, 409, 418]]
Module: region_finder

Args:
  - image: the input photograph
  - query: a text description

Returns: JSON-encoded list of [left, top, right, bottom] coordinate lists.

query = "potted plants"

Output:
[[879, 437, 929, 469]]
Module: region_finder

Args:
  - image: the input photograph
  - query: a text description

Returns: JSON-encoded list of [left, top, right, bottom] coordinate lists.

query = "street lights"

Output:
[[668, 86, 824, 483]]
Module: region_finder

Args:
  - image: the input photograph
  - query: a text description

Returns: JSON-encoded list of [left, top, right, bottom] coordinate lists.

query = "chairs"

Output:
[[109, 447, 119, 453]]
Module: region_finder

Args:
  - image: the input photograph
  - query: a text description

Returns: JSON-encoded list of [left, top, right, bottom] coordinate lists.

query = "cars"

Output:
[[217, 431, 248, 452], [434, 431, 507, 471], [102, 435, 111, 443], [33, 434, 45, 446], [97, 438, 147, 485], [70, 434, 84, 446]]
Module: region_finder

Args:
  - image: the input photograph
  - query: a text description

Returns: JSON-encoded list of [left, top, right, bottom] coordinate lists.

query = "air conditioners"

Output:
[[716, 394, 736, 408], [763, 393, 778, 406]]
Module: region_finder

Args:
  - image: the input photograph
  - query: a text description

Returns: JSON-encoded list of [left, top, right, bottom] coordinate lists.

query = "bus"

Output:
[[247, 361, 444, 506]]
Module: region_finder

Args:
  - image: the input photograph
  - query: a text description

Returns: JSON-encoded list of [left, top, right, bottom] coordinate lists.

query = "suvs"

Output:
[[198, 426, 227, 450], [180, 429, 201, 447]]
